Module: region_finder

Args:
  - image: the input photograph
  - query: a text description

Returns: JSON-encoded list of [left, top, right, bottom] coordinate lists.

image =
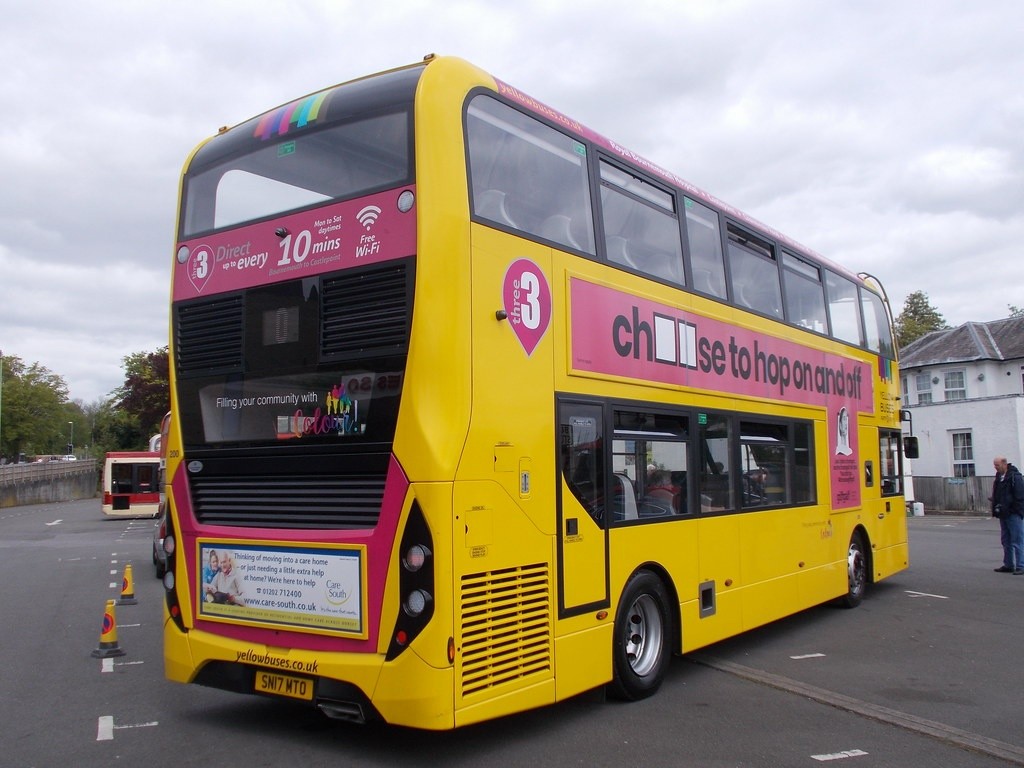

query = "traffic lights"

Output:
[[68, 444, 73, 454]]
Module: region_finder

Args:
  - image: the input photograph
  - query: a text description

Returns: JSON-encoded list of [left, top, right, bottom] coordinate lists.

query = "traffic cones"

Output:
[[114, 563, 139, 604], [90, 600, 128, 658]]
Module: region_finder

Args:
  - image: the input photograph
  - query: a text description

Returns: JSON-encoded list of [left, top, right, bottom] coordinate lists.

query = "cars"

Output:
[[60, 455, 77, 461]]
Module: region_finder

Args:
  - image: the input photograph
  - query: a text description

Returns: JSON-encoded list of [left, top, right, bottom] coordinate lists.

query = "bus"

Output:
[[153, 51, 920, 733], [99, 450, 161, 518]]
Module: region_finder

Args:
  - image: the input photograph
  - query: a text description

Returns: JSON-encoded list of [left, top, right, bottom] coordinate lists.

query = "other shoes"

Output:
[[1013, 568, 1024, 575], [994, 565, 1014, 572]]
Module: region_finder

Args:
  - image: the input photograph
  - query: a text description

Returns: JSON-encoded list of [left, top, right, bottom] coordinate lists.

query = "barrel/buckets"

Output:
[[913, 501, 924, 516]]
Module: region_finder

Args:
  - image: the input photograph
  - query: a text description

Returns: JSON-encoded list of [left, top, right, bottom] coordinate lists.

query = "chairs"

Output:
[[471, 186, 783, 320], [603, 473, 810, 521]]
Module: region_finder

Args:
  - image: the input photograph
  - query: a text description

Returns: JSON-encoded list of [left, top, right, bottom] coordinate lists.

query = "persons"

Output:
[[647, 465, 682, 515], [839, 407, 848, 445], [714, 462, 724, 473], [991, 455, 1024, 575], [203, 550, 245, 607]]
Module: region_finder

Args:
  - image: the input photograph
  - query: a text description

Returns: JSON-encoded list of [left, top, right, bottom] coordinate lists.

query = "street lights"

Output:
[[68, 422, 73, 454]]
